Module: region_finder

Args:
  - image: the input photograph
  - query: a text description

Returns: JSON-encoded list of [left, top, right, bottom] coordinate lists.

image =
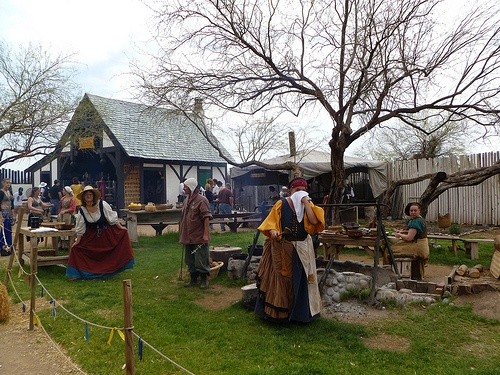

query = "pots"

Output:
[[248, 245, 263, 256]]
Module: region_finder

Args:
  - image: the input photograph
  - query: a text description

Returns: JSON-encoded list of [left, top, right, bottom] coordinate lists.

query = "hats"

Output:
[[76, 186, 101, 201], [39, 182, 47, 187]]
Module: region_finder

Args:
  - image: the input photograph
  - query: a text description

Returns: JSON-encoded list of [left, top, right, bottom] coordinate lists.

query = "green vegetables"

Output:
[[385, 227, 395, 236]]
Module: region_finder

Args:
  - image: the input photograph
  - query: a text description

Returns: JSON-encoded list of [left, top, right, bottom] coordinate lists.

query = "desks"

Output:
[[120, 209, 180, 236], [210, 246, 242, 272], [320, 235, 389, 267], [17, 227, 75, 268], [40, 222, 74, 249]]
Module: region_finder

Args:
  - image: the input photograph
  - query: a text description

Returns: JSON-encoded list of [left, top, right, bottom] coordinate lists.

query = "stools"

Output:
[[393, 255, 428, 280]]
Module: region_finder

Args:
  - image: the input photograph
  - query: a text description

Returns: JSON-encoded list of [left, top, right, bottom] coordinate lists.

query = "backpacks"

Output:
[[43, 187, 48, 197]]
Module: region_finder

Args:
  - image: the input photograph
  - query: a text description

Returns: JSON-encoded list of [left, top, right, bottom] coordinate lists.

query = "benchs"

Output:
[[208, 212, 245, 232], [427, 235, 479, 259]]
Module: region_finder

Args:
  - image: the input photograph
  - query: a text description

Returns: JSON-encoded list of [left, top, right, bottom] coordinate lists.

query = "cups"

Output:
[[31, 217, 43, 228], [145, 202, 156, 212], [64, 214, 71, 224]]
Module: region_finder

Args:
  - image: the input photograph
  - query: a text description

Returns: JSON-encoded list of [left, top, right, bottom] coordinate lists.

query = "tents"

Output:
[[230, 149, 388, 212]]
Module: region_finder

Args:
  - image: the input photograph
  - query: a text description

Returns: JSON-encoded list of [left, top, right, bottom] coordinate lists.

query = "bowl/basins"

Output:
[[231, 254, 248, 260], [128, 206, 143, 211], [160, 204, 173, 209], [58, 224, 73, 230]]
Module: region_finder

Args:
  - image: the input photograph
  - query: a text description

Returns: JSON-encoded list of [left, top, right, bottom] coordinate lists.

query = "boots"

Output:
[[200, 273, 209, 289], [184, 272, 198, 287]]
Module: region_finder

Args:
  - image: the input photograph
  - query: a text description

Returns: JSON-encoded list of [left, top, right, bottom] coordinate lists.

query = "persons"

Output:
[[340, 179, 354, 203], [66, 184, 135, 281], [5, 177, 104, 251], [268, 185, 289, 200], [253, 177, 325, 326], [0, 178, 16, 256], [382, 202, 429, 268], [198, 178, 234, 231], [179, 178, 213, 289]]
